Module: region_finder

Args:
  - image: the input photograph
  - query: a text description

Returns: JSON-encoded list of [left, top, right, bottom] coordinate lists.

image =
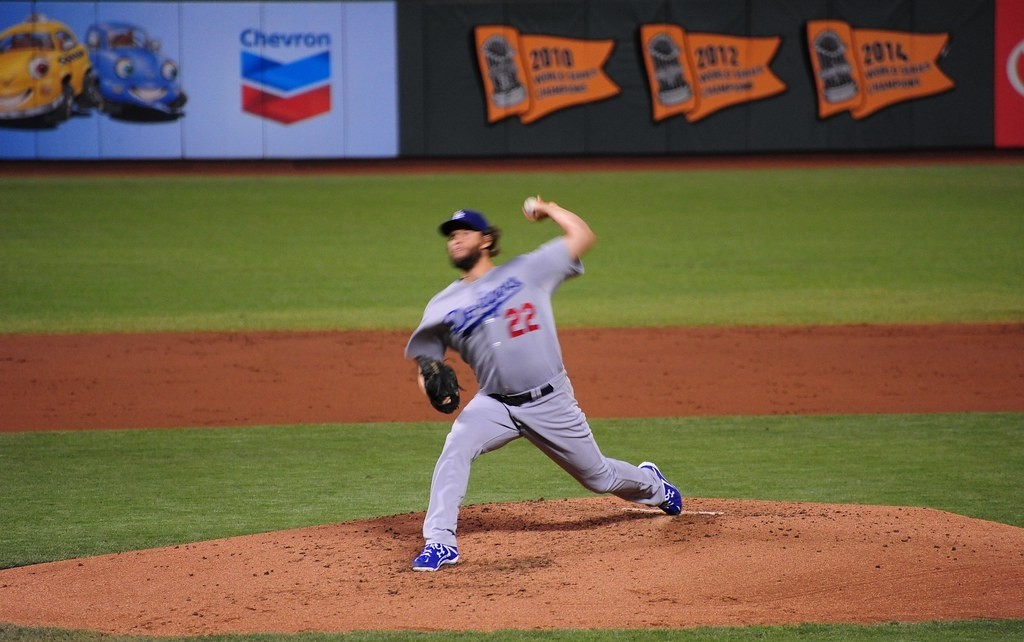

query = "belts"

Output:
[[487, 385, 553, 404]]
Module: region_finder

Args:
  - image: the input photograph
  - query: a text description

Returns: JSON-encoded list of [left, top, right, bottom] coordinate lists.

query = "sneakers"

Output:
[[638, 462, 682, 515], [413, 543, 460, 571]]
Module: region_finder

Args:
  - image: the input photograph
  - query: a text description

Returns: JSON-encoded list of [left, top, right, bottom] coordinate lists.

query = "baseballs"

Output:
[[523, 196, 537, 215]]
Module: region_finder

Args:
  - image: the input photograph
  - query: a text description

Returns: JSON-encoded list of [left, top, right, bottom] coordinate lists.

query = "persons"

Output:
[[404, 194, 682, 572]]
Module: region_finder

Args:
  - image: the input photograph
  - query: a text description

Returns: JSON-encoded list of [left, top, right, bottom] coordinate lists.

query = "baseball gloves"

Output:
[[413, 354, 460, 414]]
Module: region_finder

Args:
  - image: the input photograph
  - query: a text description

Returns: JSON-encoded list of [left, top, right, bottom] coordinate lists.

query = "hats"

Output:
[[438, 209, 489, 236]]
